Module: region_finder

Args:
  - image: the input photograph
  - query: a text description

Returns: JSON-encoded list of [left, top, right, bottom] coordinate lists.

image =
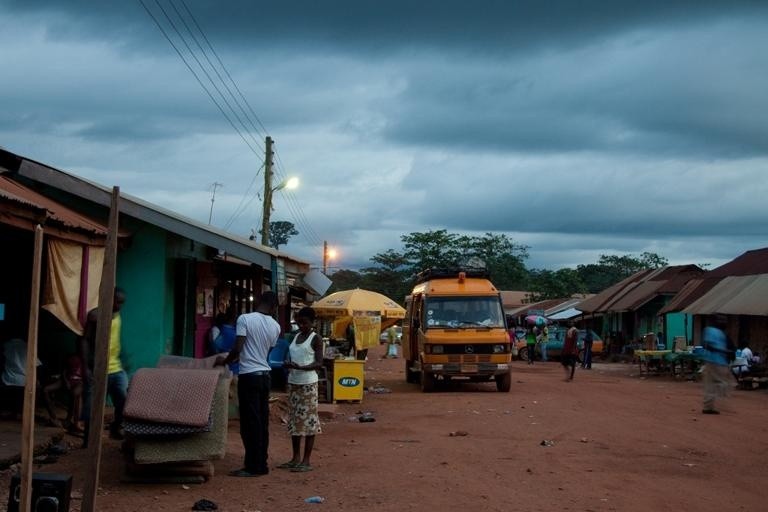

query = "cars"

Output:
[[510, 325, 604, 363], [380, 325, 403, 345]]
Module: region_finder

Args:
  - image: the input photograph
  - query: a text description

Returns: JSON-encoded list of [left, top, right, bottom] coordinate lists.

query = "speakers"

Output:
[[7, 473, 73, 512]]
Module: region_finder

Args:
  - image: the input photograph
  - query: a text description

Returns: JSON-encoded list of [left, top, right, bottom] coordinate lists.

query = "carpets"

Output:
[[109, 351, 233, 467]]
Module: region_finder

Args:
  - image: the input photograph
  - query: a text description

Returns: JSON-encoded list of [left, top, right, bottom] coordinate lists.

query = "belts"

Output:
[[250, 371, 269, 375]]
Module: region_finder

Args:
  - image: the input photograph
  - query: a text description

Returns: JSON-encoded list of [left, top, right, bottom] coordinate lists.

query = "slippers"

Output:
[[276, 461, 298, 468], [292, 465, 312, 472]]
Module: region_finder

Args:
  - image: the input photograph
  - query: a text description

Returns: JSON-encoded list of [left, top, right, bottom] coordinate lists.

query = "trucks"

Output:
[[399, 266, 514, 393]]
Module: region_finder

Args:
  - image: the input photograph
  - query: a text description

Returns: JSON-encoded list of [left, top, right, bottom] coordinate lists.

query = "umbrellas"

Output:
[[307, 287, 408, 322], [526, 312, 552, 328]]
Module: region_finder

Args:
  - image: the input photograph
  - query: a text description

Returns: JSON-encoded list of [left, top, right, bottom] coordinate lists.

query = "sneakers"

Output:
[[703, 408, 720, 415], [230, 469, 268, 477]]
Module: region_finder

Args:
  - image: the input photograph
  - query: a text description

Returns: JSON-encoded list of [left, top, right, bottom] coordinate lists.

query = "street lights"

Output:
[[263, 176, 301, 244], [323, 250, 336, 273]]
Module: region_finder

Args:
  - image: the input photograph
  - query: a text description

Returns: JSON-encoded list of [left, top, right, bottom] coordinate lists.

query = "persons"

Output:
[[224, 291, 283, 476], [208, 310, 226, 345], [702, 315, 734, 414], [507, 320, 549, 365], [655, 332, 663, 346], [82, 283, 130, 450], [338, 320, 399, 360], [561, 318, 593, 378], [276, 306, 324, 473], [729, 342, 760, 378]]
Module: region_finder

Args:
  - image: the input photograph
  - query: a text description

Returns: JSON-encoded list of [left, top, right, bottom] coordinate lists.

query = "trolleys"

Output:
[[731, 364, 768, 390]]
